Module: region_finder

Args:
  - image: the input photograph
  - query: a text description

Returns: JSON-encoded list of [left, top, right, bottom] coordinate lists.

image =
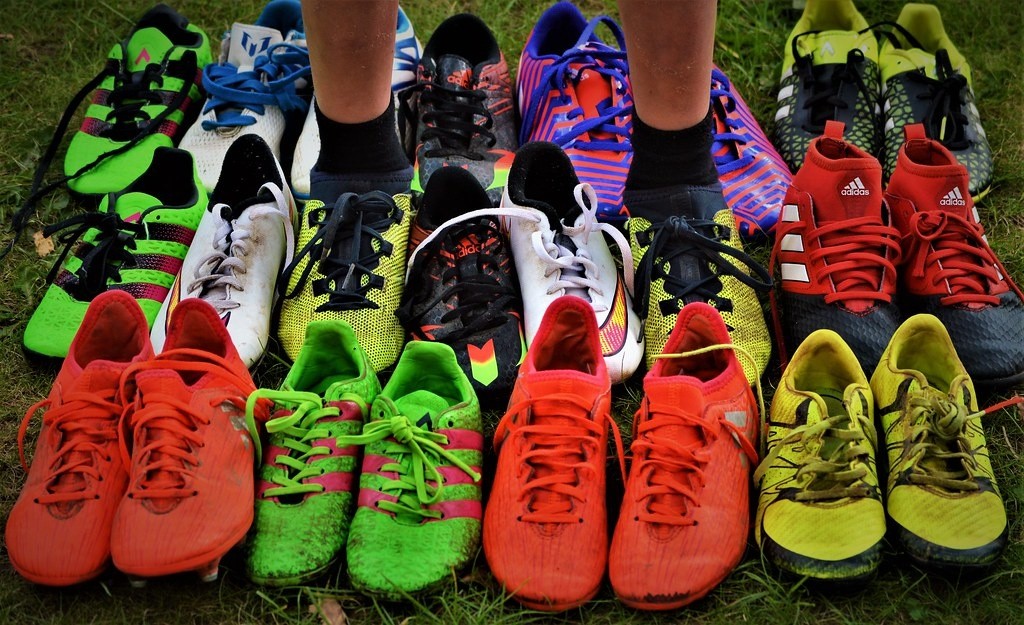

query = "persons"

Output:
[[279, 0, 773, 383]]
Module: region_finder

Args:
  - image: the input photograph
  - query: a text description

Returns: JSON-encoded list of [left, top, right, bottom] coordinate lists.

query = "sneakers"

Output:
[[398, 12, 513, 208], [620, 186, 772, 386], [150, 134, 299, 370], [516, 1, 635, 227], [335, 341, 483, 601], [111, 298, 274, 577], [6, 290, 153, 586], [882, 124, 1024, 390], [868, 313, 1023, 573], [652, 329, 889, 580], [482, 295, 611, 611], [768, 120, 904, 375], [23, 146, 210, 373], [711, 64, 794, 239], [245, 320, 382, 587], [774, 0, 880, 176], [177, 0, 311, 194], [403, 141, 646, 387], [608, 302, 760, 609], [279, 190, 412, 375], [405, 166, 527, 404], [4, 4, 214, 264], [290, 5, 424, 200], [878, 3, 995, 201]]
